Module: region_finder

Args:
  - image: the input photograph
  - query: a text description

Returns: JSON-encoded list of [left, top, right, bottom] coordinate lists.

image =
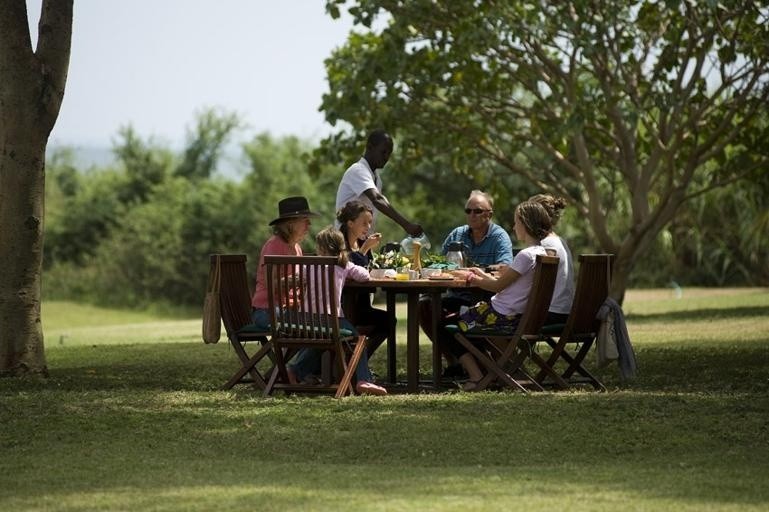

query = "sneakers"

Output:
[[286, 365, 297, 386], [356, 382, 388, 396], [454, 366, 484, 383]]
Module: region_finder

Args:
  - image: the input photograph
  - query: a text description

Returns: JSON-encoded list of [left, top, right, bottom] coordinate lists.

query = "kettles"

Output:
[[380, 243, 403, 254], [446, 241, 472, 267]]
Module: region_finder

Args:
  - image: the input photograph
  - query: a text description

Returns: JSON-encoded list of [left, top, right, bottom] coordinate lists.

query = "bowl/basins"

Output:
[[370, 269, 395, 279], [429, 269, 441, 277]]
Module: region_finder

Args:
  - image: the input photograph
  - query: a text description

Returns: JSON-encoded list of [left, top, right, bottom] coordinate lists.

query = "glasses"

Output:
[[464, 208, 492, 214]]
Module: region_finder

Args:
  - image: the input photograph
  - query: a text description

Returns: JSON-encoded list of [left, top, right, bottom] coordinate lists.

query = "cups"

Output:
[[410, 270, 419, 280], [423, 268, 432, 278], [395, 266, 409, 281]]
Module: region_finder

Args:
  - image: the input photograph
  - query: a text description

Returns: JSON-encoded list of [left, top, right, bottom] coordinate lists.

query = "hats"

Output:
[[268, 196, 322, 226]]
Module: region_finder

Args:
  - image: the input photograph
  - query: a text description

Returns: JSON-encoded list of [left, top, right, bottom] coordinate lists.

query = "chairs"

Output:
[[211, 255, 281, 392], [527, 254, 616, 393], [455, 254, 560, 392], [261, 255, 368, 398]]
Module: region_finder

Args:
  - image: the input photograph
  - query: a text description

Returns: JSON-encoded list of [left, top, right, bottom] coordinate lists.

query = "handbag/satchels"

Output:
[[598, 254, 618, 367], [202, 253, 222, 345]]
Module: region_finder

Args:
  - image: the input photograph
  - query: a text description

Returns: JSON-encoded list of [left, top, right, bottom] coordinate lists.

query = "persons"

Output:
[[335, 201, 397, 386], [277, 227, 387, 396], [335, 130, 423, 261], [437, 201, 552, 392], [417, 190, 514, 382], [527, 194, 575, 330], [253, 198, 321, 390]]
